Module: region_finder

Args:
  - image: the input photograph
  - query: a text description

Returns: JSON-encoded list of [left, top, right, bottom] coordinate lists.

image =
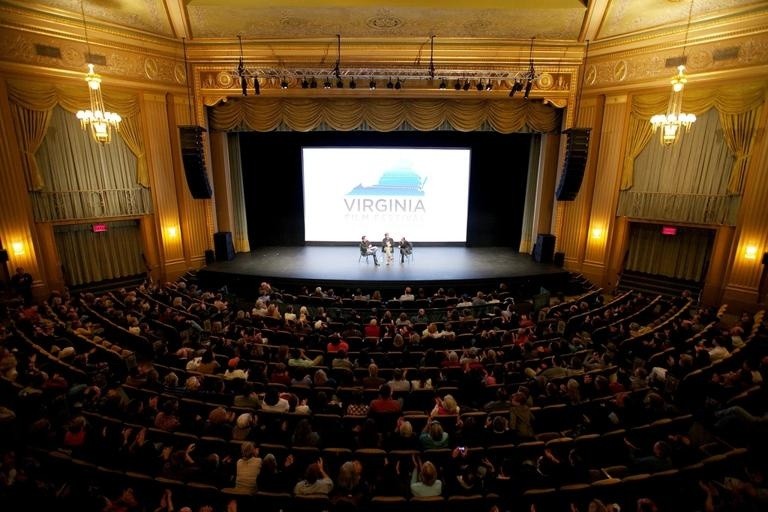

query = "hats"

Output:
[[227, 356, 241, 367]]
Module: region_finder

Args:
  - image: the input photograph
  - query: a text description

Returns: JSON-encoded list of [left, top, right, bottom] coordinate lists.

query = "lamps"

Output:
[[76, 0, 122, 146], [650, 0, 697, 150]]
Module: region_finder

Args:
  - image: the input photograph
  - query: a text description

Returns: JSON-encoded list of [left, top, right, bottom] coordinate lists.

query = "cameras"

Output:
[[459, 446, 464, 452]]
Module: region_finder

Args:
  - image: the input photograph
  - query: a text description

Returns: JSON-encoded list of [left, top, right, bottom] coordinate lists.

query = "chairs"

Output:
[[359, 243, 413, 264]]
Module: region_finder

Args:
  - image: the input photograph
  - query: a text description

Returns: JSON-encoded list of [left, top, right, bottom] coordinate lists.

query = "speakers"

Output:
[[205, 249, 213, 265], [176, 124, 212, 199], [534, 234, 556, 263], [555, 128, 593, 201], [214, 232, 235, 261], [554, 252, 564, 266]]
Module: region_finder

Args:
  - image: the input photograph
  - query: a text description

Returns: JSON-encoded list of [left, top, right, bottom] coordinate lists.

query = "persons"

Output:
[[360, 234, 382, 266], [1, 277, 768, 511], [381, 233, 395, 266], [398, 235, 414, 263]]
